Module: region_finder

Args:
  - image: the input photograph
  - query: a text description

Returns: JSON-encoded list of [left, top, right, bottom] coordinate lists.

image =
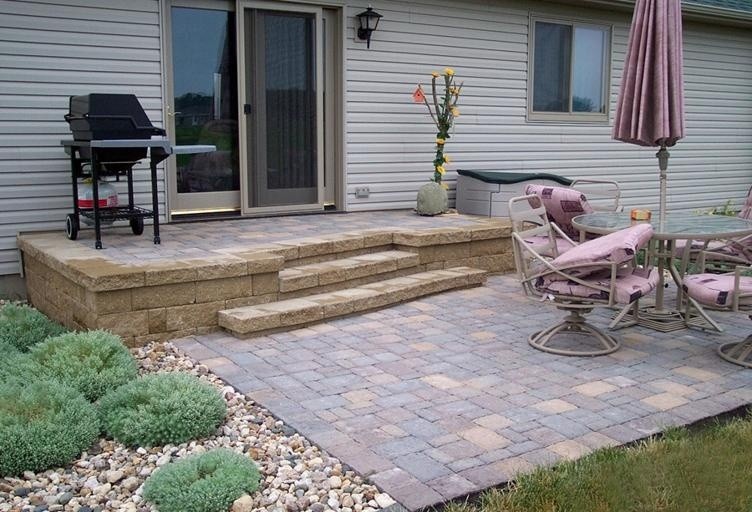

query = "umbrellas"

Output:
[[610, 0, 686, 312]]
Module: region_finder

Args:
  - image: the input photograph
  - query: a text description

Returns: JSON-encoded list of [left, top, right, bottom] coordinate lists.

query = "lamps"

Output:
[[357, 5, 384, 49]]
